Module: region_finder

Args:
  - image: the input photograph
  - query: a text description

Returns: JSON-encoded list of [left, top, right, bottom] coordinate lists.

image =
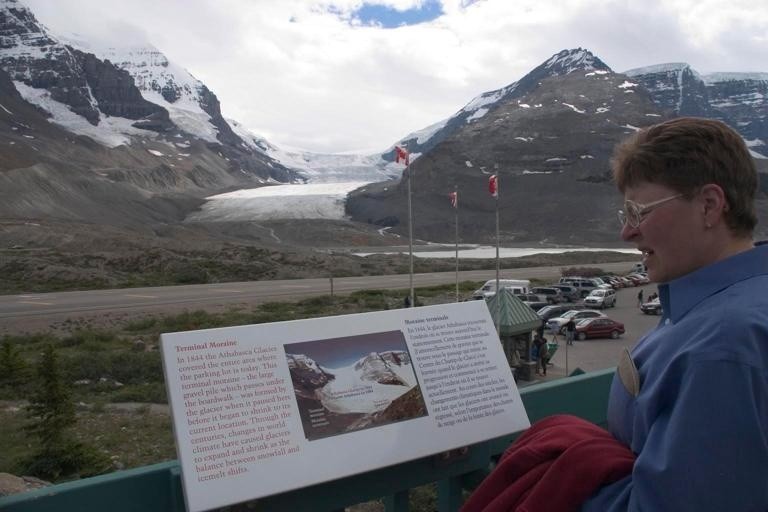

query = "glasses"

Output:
[[615, 188, 729, 229]]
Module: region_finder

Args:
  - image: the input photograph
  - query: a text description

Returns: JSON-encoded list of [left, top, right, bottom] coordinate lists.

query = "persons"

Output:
[[562, 316, 577, 345], [550, 321, 560, 344], [638, 289, 644, 305], [652, 292, 657, 299], [648, 296, 652, 302], [512, 347, 522, 367], [459, 115, 768, 512], [532, 334, 554, 376]]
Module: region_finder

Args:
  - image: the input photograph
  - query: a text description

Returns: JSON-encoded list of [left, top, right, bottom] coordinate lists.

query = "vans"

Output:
[[581, 289, 618, 309], [536, 305, 585, 329]]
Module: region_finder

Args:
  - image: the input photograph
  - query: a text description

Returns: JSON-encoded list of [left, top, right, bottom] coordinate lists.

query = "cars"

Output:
[[522, 301, 549, 313], [499, 285, 527, 296], [561, 316, 626, 339], [546, 309, 609, 336], [529, 260, 651, 304], [639, 296, 666, 316]]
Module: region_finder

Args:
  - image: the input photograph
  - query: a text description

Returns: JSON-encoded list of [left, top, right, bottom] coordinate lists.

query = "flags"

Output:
[[395, 145, 408, 165], [489, 175, 497, 197], [449, 192, 456, 208]]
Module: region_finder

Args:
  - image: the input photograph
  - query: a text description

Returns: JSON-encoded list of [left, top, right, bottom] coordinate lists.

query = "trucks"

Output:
[[471, 279, 533, 300]]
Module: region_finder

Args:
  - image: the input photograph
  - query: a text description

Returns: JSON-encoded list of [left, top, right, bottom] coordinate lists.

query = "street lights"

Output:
[[476, 162, 501, 346]]
